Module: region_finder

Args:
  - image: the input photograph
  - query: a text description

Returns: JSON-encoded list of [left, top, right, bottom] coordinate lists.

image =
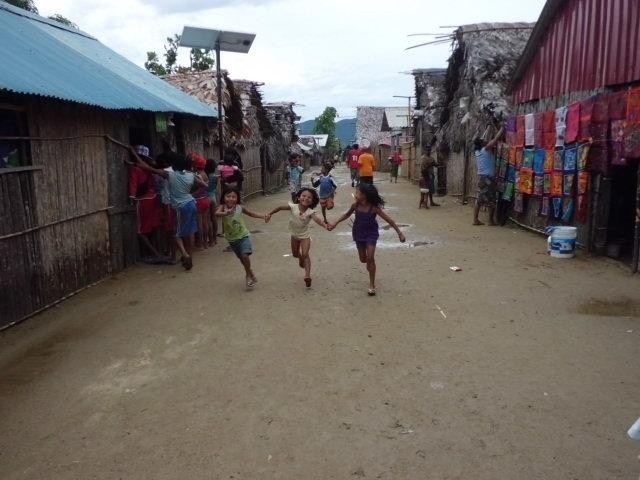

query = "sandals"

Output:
[[368, 287, 376, 295], [181, 254, 192, 270], [299, 256, 304, 268], [245, 272, 258, 288], [304, 277, 312, 287]]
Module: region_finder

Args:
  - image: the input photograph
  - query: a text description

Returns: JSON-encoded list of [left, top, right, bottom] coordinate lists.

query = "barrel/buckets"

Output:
[[550, 226, 577, 259]]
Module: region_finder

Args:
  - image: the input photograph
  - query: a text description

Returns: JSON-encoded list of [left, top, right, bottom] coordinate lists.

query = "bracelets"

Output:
[[487, 123, 493, 126], [397, 231, 402, 235]]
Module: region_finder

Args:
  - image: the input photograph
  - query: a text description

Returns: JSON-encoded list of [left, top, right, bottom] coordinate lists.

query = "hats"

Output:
[[135, 145, 153, 160]]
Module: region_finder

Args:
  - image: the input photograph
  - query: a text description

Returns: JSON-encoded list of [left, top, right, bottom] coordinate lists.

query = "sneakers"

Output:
[[145, 256, 159, 263]]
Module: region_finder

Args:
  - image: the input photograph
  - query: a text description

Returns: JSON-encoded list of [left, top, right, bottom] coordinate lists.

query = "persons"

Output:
[[346, 143, 362, 188], [356, 144, 377, 184], [420, 145, 442, 206], [326, 182, 407, 296], [472, 115, 507, 226], [124, 136, 245, 272], [262, 186, 334, 289], [387, 151, 401, 185], [213, 186, 272, 291], [284, 153, 306, 204], [418, 168, 430, 209], [310, 162, 336, 223]]
